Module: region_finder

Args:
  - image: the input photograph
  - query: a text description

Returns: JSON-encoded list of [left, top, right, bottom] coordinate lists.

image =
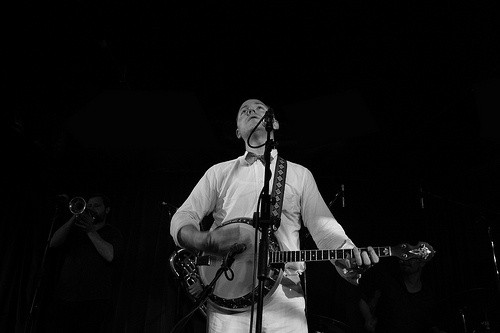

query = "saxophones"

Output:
[[170, 248, 208, 317]]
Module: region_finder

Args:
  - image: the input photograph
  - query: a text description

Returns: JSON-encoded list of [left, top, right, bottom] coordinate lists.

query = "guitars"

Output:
[[197, 217, 434, 312]]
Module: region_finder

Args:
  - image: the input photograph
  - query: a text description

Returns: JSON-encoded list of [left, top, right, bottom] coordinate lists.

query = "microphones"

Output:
[[270, 108, 280, 130]]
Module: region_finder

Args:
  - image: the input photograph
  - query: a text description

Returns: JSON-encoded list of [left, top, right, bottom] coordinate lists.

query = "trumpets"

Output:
[[68, 196, 98, 224]]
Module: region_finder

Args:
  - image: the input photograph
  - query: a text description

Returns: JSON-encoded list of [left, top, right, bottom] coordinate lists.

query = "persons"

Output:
[[170, 99, 379, 333], [358, 259, 456, 333], [43, 189, 123, 333]]
